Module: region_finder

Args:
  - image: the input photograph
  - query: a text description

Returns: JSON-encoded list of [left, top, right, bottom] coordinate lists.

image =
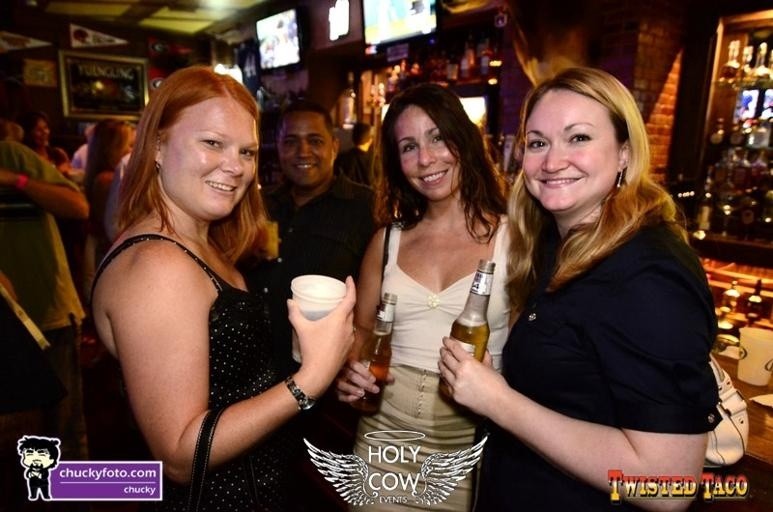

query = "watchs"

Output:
[[284, 376, 316, 412]]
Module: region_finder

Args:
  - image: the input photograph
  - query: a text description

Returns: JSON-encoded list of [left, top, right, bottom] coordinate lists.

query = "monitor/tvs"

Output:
[[255, 8, 302, 72], [362, 0, 439, 45]]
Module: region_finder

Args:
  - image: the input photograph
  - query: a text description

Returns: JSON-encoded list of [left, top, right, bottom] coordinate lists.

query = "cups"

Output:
[[288, 272, 353, 366], [735, 326, 773, 387]]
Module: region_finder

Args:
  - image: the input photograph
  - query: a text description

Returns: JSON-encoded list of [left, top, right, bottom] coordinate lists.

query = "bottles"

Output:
[[719, 279, 741, 314], [352, 290, 397, 413], [744, 279, 764, 321], [691, 39, 773, 239], [387, 35, 496, 92], [435, 259, 495, 392]]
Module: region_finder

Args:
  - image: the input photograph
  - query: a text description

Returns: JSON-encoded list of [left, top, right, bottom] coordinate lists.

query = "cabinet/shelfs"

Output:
[[682, 11, 773, 254]]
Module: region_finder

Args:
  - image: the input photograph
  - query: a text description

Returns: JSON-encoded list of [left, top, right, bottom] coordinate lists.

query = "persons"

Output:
[[334, 122, 377, 189], [79, 65, 357, 512], [436, 67, 723, 512], [243, 100, 377, 486], [0, 105, 143, 470], [344, 81, 524, 511]]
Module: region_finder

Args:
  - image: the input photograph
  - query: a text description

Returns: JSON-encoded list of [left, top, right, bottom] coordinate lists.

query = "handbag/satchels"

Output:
[[705, 352, 749, 468]]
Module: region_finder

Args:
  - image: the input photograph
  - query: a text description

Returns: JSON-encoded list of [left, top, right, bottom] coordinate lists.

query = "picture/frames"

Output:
[[55, 48, 151, 126]]
